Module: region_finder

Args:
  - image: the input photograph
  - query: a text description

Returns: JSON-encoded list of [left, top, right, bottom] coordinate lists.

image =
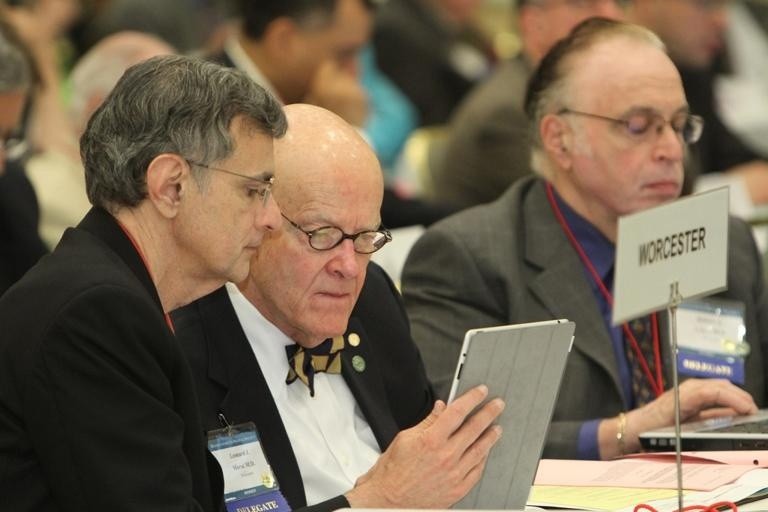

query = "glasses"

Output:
[[552, 103, 706, 147], [274, 201, 394, 254], [182, 156, 275, 206]]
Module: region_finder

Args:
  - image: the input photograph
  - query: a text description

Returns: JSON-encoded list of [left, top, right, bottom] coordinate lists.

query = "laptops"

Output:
[[638, 408, 768, 450]]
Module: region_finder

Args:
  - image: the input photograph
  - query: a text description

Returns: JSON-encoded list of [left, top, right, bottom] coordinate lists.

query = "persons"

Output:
[[1, 54, 509, 510], [400, 14, 768, 459], [168, 102, 434, 512]]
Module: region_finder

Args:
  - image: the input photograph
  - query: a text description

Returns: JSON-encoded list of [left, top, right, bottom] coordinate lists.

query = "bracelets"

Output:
[[617, 412, 626, 456]]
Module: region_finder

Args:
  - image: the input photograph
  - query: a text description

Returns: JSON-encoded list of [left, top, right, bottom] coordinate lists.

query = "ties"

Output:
[[621, 315, 664, 407]]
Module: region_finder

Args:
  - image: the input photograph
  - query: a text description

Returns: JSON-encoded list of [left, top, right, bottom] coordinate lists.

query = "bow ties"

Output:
[[281, 340, 348, 391]]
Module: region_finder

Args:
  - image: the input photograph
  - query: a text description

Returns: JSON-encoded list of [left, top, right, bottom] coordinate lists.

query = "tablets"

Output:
[[445, 317, 577, 510]]
